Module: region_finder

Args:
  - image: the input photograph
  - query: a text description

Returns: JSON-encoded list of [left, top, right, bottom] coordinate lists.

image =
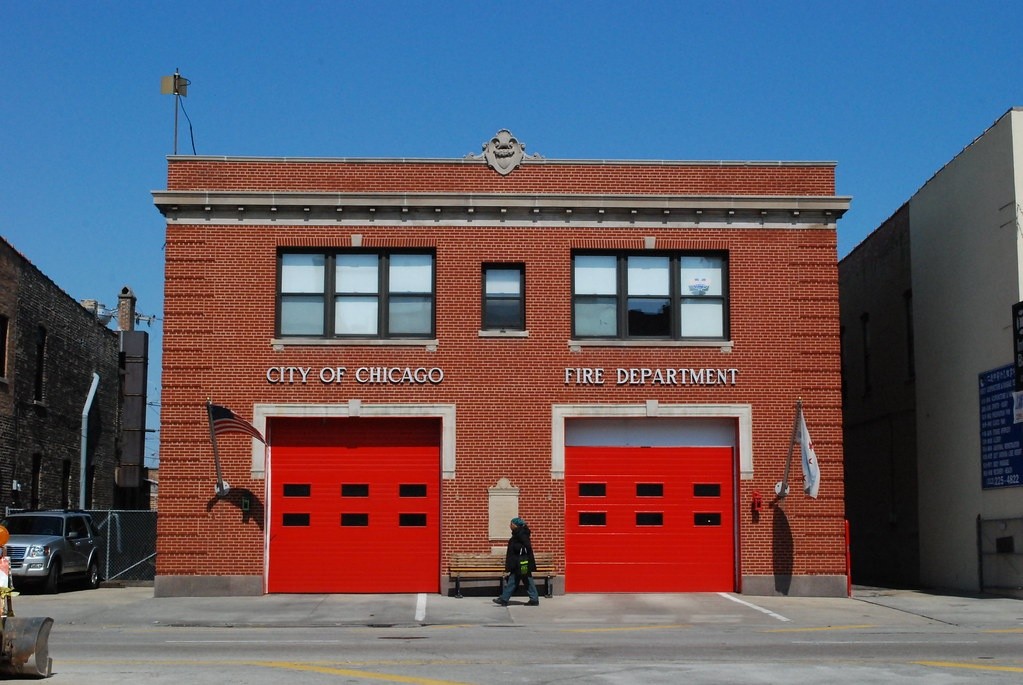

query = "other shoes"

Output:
[[524, 600, 539, 606], [493, 597, 508, 606]]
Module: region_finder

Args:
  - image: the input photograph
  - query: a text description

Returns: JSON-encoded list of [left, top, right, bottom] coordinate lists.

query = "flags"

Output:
[[796, 408, 820, 499], [210, 405, 268, 448]]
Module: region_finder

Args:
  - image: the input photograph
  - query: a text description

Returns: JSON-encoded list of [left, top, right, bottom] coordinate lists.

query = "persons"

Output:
[[493, 518, 539, 606]]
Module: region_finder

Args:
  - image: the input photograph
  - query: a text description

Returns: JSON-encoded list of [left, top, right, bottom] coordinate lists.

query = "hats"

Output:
[[511, 517, 525, 527]]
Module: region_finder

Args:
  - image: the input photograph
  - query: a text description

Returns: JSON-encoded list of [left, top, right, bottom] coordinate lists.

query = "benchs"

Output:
[[448, 551, 557, 599]]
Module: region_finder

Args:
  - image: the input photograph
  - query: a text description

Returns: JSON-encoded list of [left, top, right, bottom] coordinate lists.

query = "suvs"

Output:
[[0, 511, 100, 596]]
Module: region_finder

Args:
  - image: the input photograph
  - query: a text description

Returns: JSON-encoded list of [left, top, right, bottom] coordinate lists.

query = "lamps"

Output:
[[168, 203, 836, 220]]
